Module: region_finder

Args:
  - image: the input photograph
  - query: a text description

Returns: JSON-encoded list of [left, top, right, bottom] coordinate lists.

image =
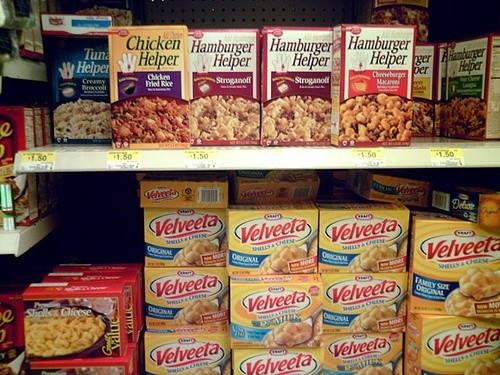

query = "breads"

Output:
[[171, 238, 500, 375]]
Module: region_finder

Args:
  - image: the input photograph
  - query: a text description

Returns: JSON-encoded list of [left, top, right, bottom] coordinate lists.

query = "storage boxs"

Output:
[[0, 0, 500, 375]]
